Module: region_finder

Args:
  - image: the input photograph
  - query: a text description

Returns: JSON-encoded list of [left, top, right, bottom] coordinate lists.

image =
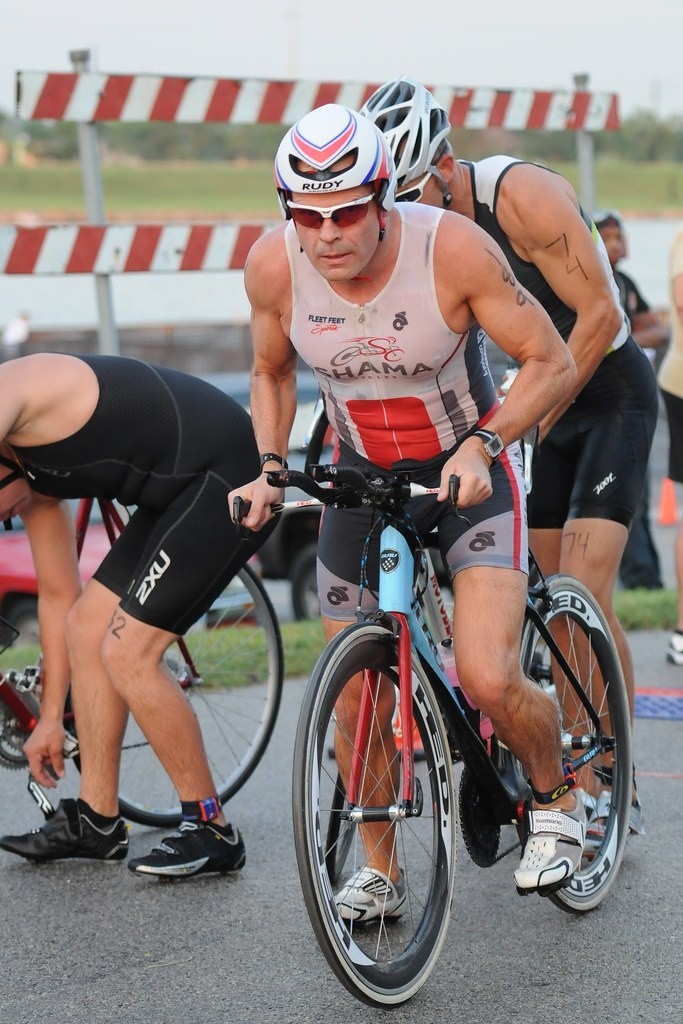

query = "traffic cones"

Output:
[[658, 477, 678, 525]]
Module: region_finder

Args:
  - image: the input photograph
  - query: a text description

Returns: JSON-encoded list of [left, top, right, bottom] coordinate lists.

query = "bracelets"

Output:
[[260, 453, 288, 472]]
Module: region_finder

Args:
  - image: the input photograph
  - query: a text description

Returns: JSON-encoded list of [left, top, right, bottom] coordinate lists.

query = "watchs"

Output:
[[473, 429, 504, 467]]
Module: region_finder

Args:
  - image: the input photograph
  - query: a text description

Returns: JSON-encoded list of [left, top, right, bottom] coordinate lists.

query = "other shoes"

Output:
[[667, 632, 683, 666]]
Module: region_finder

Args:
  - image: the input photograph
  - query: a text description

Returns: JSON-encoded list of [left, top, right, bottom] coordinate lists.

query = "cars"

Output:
[[191, 346, 674, 623], [1, 513, 261, 644]]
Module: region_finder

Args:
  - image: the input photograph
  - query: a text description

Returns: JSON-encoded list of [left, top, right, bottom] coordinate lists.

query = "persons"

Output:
[[0, 353, 286, 884], [357, 74, 663, 862], [592, 209, 669, 591], [226, 102, 587, 930], [656, 227, 683, 667]]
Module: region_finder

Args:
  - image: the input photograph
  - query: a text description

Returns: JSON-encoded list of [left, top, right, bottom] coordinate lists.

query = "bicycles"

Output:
[[233, 459, 634, 1011], [0, 498, 285, 831]]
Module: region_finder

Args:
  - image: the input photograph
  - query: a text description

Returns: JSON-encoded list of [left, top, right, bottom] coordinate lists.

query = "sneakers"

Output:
[[513, 789, 588, 888], [334, 867, 408, 922], [571, 789, 643, 857], [128, 821, 246, 877], [0, 798, 129, 865]]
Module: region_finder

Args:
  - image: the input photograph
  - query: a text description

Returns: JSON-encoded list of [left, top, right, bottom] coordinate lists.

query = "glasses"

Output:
[[395, 172, 432, 202], [287, 192, 375, 229]]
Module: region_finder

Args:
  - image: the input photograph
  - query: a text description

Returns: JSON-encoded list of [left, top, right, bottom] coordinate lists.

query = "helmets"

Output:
[[274, 102, 398, 220], [359, 76, 451, 187]]
